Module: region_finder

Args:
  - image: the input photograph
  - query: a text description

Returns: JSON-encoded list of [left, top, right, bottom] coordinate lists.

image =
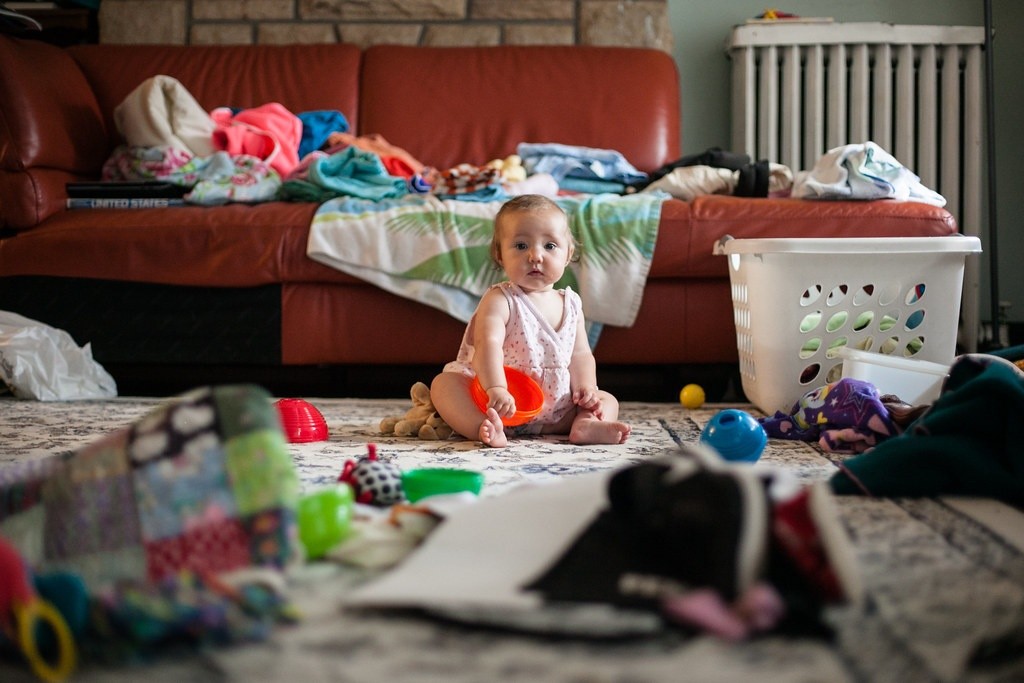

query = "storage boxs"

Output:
[[839, 347, 951, 406], [711, 232, 982, 419]]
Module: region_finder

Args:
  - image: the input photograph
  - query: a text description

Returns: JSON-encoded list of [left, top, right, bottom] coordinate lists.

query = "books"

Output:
[[66, 178, 191, 197], [64, 197, 192, 209]]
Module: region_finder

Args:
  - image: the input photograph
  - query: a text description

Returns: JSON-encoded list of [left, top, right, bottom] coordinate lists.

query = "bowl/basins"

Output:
[[403, 468, 482, 503], [275, 398, 329, 443], [472, 369, 545, 426]]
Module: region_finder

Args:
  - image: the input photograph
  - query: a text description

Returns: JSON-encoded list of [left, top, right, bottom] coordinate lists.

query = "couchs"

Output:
[[2, 34, 961, 394]]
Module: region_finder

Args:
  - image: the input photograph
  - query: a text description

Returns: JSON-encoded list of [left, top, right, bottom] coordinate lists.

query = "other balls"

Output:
[[680, 384, 705, 408]]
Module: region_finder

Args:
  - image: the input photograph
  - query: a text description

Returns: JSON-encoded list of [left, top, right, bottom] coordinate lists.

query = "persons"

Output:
[[430, 194, 634, 447]]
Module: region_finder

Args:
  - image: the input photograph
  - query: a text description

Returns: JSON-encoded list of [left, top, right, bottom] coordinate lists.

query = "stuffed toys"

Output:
[[380, 381, 456, 440]]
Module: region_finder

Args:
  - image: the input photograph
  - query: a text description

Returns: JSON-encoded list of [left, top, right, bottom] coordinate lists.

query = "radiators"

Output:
[[726, 24, 988, 345]]
